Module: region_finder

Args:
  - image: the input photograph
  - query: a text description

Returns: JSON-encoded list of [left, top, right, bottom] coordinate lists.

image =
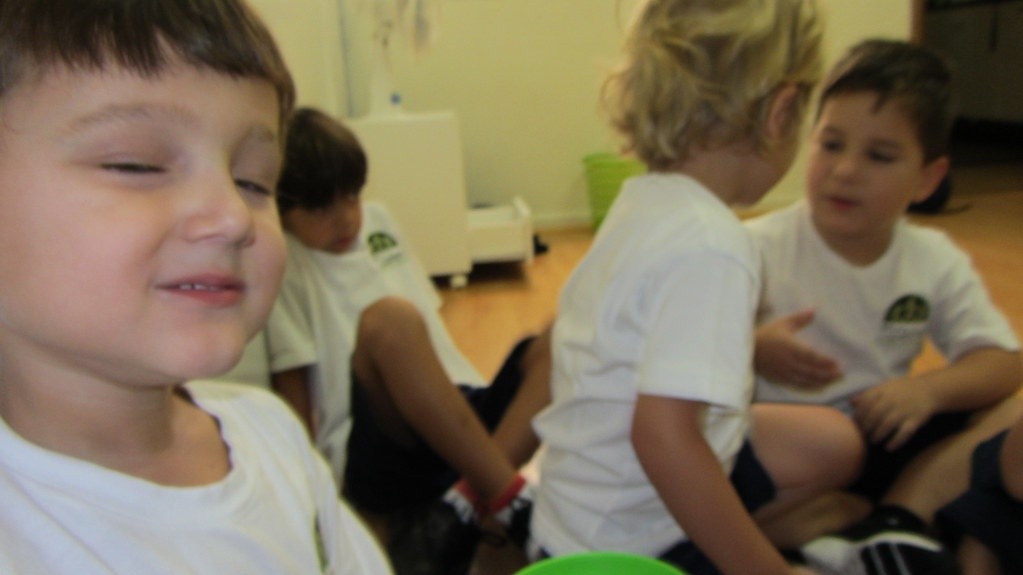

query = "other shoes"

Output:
[[385, 497, 481, 575], [501, 497, 545, 561], [800, 503, 949, 575]]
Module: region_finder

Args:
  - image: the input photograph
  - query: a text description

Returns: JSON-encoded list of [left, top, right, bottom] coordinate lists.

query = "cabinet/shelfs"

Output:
[[350, 107, 537, 289]]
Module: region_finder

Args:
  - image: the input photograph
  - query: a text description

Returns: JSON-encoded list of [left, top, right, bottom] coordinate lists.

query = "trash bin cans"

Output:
[[585, 154, 648, 229]]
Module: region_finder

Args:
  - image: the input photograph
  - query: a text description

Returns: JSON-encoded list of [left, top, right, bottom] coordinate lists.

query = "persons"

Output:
[[262, 108, 550, 575], [743, 41, 1023, 575], [528, 1, 862, 575], [0, 0, 393, 575]]
